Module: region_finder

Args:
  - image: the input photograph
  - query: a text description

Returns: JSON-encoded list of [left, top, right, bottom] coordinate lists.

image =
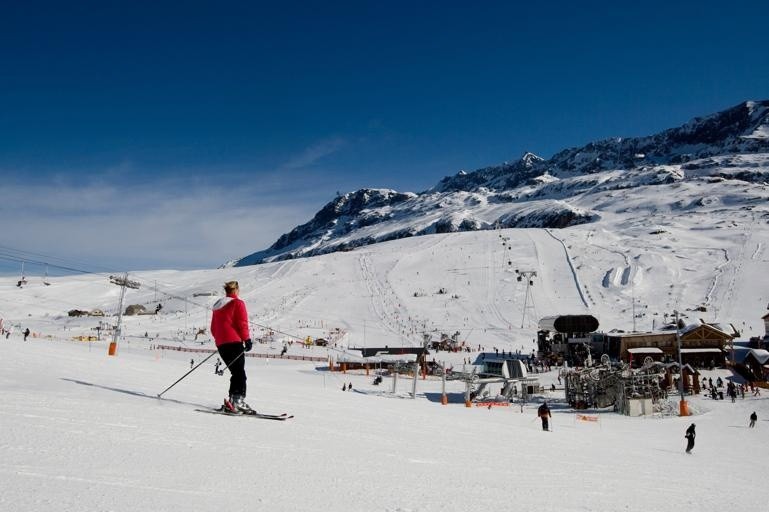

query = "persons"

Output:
[[536, 399, 551, 432], [189, 359, 195, 368], [685, 422, 697, 454], [213, 357, 222, 374], [593, 400, 598, 410], [749, 412, 757, 428], [22, 327, 30, 343], [210, 281, 254, 415], [707, 378, 761, 404]]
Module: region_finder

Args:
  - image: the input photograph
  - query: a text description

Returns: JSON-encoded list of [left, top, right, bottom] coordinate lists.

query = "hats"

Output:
[[224, 281, 238, 289]]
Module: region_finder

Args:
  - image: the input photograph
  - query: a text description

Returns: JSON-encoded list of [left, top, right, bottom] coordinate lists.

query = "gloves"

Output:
[[245, 339, 252, 351]]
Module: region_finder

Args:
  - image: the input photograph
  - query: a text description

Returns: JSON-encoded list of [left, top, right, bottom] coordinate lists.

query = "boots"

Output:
[[229, 395, 255, 414]]
